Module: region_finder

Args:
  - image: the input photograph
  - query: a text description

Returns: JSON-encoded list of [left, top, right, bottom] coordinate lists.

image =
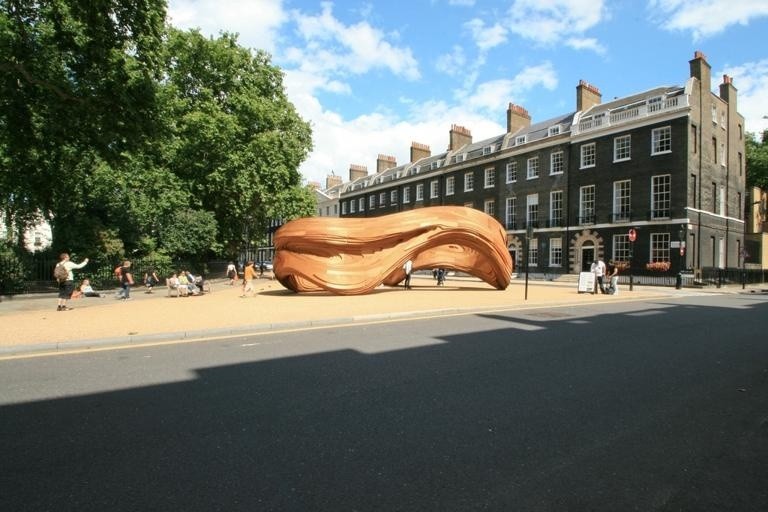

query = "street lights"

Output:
[[524, 226, 533, 300], [674, 230, 685, 290]]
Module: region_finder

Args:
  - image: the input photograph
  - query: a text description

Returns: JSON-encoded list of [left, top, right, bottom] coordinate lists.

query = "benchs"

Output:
[[166, 278, 212, 298]]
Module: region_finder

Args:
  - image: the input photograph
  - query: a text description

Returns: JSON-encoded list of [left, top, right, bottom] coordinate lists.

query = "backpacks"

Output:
[[54, 265, 69, 280]]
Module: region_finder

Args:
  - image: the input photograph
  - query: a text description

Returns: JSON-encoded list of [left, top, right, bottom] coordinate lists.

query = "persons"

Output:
[[604, 261, 619, 296], [142, 265, 159, 295], [79, 278, 106, 298], [436, 270, 444, 286], [239, 260, 260, 297], [184, 270, 203, 295], [673, 270, 682, 289], [402, 259, 413, 289], [589, 258, 604, 295], [430, 268, 438, 280], [116, 260, 134, 301], [226, 260, 237, 287], [177, 270, 200, 296], [53, 252, 89, 311], [167, 271, 184, 298]]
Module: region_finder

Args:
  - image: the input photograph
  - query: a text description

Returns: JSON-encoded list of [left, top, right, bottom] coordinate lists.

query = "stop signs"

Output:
[[679, 246, 684, 257], [628, 229, 636, 242]]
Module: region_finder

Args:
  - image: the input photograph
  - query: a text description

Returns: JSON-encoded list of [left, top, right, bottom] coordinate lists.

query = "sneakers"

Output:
[[57, 305, 73, 311]]
